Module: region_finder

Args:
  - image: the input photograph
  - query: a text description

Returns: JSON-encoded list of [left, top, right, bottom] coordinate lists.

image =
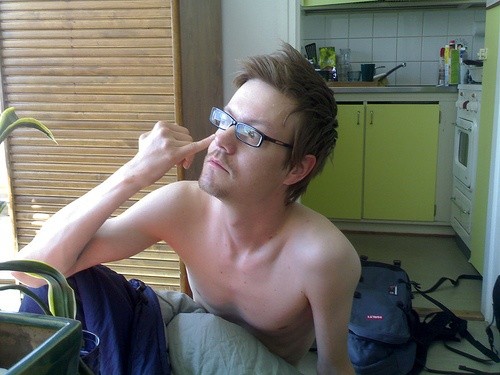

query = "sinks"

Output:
[[385, 85, 436, 87]]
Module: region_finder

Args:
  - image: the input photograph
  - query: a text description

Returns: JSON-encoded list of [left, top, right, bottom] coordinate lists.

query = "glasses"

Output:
[[208, 105, 294, 151]]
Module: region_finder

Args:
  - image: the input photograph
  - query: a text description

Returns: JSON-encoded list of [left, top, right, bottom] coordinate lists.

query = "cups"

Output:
[[313, 68, 336, 82], [361, 63, 375, 82], [347, 71, 361, 82]]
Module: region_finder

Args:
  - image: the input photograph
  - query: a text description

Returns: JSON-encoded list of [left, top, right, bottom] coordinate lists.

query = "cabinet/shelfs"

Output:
[[299, 103, 439, 222]]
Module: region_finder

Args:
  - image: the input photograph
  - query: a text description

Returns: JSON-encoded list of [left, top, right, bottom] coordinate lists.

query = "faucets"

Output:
[[378, 62, 406, 82]]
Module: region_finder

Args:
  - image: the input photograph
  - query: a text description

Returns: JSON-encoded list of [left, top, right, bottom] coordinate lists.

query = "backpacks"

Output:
[[348, 255, 423, 375]]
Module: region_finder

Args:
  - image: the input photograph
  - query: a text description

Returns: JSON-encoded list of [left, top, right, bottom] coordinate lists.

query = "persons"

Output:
[[10, 40, 361, 375]]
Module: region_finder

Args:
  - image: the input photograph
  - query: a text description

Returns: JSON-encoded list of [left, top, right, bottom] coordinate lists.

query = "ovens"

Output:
[[450, 88, 481, 260]]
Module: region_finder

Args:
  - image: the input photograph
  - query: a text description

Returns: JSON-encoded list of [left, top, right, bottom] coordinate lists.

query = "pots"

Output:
[[463, 58, 483, 83]]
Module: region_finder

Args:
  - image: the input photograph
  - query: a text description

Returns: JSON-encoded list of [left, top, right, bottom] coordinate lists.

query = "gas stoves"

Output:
[[456, 79, 483, 88]]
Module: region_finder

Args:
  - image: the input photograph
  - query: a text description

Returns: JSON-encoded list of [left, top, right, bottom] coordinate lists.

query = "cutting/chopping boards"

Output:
[[326, 79, 385, 87]]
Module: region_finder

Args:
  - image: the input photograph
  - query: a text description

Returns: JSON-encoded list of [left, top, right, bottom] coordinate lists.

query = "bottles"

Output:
[[438, 40, 469, 86], [337, 49, 351, 82]]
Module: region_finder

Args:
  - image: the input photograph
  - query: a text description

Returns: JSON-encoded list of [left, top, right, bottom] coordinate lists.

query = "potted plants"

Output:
[[1, 104, 82, 375]]
